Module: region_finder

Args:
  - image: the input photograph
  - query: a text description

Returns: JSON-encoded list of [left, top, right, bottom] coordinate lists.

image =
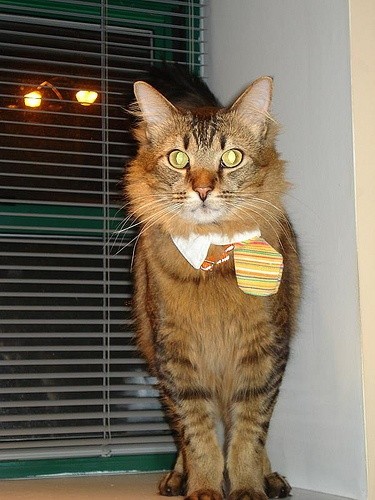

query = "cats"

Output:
[[120, 73, 304, 500]]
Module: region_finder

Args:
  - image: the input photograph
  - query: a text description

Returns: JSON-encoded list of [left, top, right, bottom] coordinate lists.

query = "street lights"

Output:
[[17, 76, 102, 164]]
